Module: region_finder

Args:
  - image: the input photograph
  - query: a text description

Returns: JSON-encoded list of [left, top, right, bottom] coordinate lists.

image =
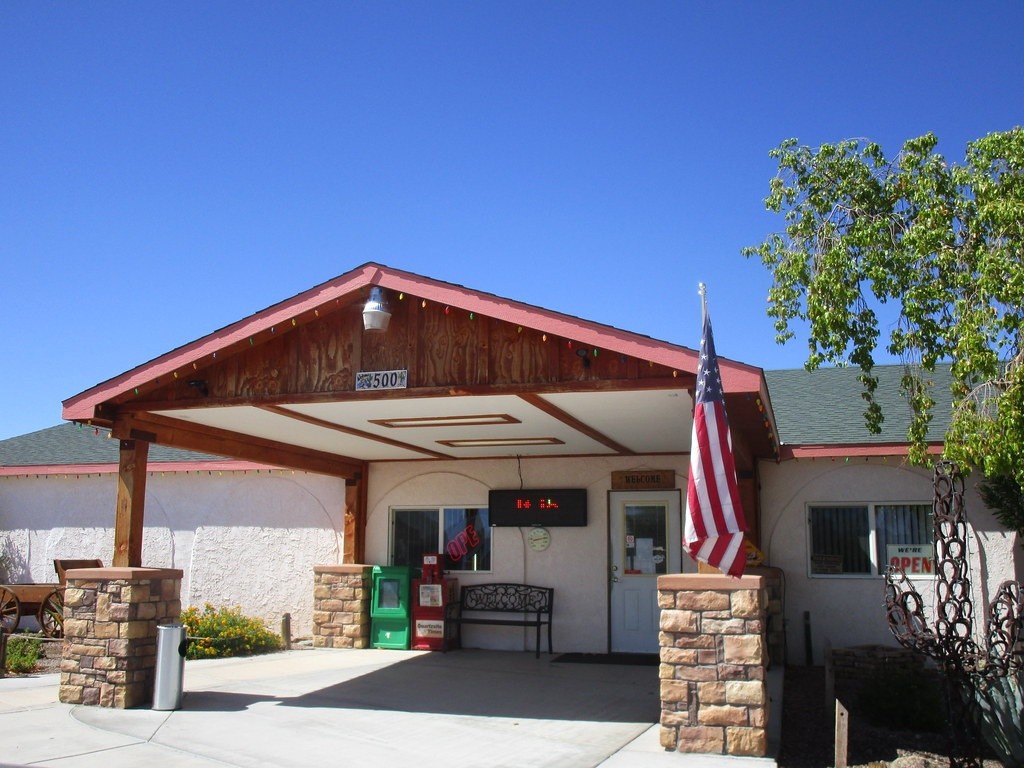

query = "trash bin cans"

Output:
[[150, 626, 187, 712]]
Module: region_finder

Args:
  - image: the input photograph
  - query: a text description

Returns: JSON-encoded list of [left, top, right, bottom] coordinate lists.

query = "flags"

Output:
[[682, 302, 767, 580]]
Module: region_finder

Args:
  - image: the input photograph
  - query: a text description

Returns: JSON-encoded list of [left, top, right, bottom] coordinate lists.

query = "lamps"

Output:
[[362, 288, 394, 332]]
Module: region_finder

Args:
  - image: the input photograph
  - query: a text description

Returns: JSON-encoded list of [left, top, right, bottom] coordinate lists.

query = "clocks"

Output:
[[528, 528, 551, 552]]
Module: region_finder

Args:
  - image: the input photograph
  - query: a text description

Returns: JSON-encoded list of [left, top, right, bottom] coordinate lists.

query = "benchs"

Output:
[[447, 583, 554, 658]]
